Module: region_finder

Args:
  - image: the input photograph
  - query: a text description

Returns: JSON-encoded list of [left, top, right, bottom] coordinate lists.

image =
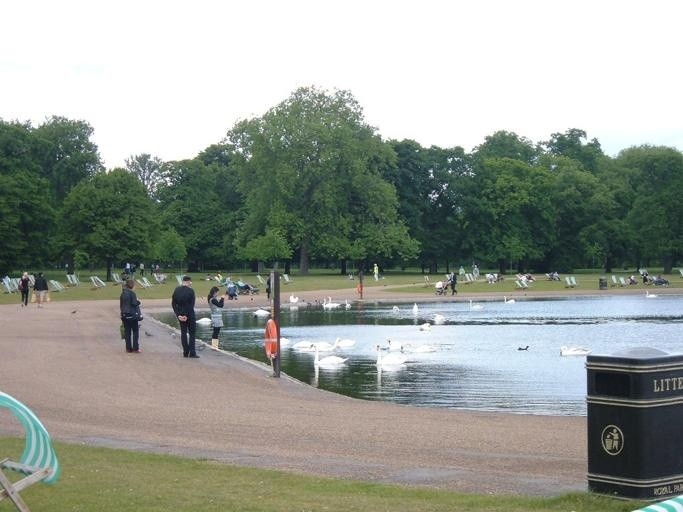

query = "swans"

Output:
[[195, 317, 213, 322], [559, 345, 593, 355], [373, 337, 413, 367], [289, 294, 299, 302], [412, 302, 419, 313], [469, 299, 484, 312], [502, 294, 515, 306], [645, 290, 657, 298], [418, 321, 431, 331], [289, 336, 355, 367], [251, 309, 271, 317], [321, 296, 353, 311]]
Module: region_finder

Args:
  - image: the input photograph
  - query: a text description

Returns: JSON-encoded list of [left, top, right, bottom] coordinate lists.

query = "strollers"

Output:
[[435, 281, 448, 295], [222, 284, 237, 300]]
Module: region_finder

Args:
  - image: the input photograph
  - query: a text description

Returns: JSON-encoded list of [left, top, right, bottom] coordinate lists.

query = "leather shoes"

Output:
[[183, 351, 188, 356], [189, 354, 199, 358]]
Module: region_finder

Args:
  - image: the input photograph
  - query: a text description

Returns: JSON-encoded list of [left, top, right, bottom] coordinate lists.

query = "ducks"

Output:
[[517, 345, 530, 351]]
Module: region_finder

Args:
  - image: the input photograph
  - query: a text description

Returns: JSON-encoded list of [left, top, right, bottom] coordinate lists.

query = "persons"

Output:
[[373, 264, 379, 281], [207, 286, 225, 349], [33, 272, 49, 308], [172, 276, 201, 358], [19, 271, 30, 307], [473, 264, 560, 289], [266, 277, 271, 298], [125, 261, 160, 277], [459, 266, 465, 281], [206, 270, 254, 300], [120, 280, 144, 353], [629, 272, 669, 286], [448, 271, 457, 295]]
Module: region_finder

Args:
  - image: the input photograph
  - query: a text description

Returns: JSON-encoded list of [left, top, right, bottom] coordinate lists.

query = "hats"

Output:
[[182, 276, 192, 281]]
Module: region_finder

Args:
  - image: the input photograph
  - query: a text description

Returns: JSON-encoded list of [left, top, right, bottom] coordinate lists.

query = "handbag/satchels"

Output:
[[30, 290, 37, 303], [120, 321, 126, 339]]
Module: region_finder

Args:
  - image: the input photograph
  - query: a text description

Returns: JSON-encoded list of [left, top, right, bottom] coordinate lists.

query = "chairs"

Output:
[[423, 273, 638, 289], [0, 391, 59, 512], [678, 270, 683, 278], [0, 269, 292, 299], [639, 270, 656, 281]]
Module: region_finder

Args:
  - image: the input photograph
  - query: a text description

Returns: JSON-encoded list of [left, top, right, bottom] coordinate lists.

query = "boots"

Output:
[[212, 338, 220, 352]]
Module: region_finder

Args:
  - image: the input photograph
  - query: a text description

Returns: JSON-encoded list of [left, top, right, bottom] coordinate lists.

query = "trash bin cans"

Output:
[[585, 347, 683, 502], [600, 278, 607, 290]]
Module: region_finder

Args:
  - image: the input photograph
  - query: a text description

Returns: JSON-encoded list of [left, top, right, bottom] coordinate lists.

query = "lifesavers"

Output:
[[266, 319, 277, 358], [357, 284, 361, 292]]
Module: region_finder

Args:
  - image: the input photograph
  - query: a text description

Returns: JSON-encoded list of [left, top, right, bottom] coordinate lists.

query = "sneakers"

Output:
[[133, 349, 141, 353], [125, 348, 132, 352]]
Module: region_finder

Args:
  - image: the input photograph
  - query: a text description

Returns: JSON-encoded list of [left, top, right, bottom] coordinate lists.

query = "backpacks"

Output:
[[17, 281, 23, 290]]
